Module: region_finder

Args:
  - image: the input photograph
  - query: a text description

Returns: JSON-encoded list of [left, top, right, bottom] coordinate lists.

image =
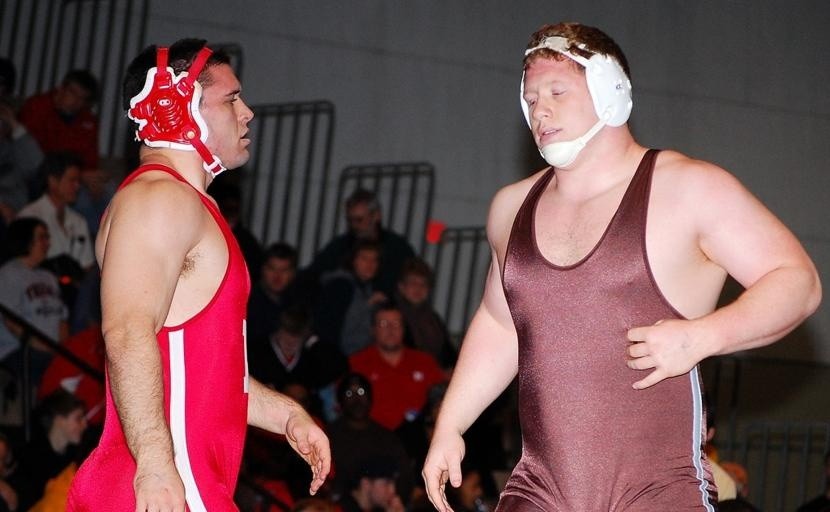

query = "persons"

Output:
[[698, 407, 754, 509], [2, 36, 332, 512], [240, 189, 519, 510], [420, 21, 822, 510]]
